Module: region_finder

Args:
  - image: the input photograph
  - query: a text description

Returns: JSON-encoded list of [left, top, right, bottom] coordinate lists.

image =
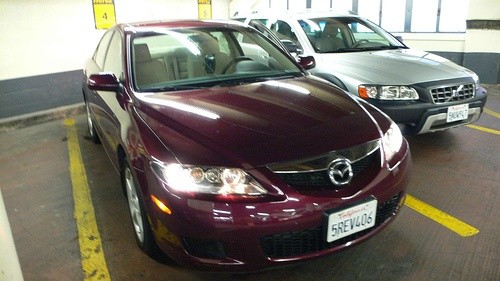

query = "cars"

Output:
[[78, 18, 412, 274]]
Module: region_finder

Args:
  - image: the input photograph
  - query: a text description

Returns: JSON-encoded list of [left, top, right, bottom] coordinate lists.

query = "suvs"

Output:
[[220, 10, 489, 137]]
